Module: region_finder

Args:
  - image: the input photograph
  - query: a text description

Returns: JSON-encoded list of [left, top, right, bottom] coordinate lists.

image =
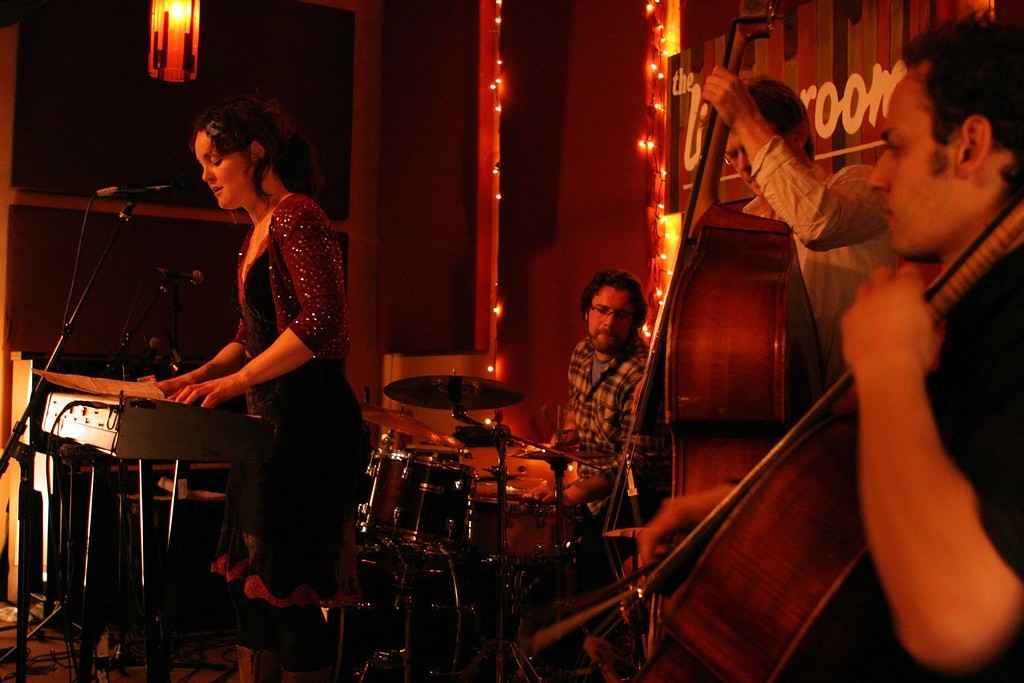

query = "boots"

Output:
[[235, 645, 277, 683]]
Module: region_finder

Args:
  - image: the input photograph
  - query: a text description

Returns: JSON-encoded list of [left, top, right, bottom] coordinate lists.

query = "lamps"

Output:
[[149, 0, 200, 82]]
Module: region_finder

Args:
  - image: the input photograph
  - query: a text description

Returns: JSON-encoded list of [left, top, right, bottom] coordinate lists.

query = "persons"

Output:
[[524, 268, 671, 674], [636, 13, 1023, 682], [701, 62, 896, 392], [157, 97, 366, 683]]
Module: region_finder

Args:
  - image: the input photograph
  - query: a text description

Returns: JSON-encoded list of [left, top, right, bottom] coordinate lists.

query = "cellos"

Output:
[[598, 0, 814, 662], [574, 186, 1024, 683]]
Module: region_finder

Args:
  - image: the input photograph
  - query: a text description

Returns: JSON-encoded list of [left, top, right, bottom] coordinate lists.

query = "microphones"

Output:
[[96, 179, 198, 197], [146, 336, 161, 366], [162, 269, 204, 286]]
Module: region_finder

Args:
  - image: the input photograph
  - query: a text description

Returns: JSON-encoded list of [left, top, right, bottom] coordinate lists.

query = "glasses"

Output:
[[589, 302, 634, 320], [723, 145, 746, 165]]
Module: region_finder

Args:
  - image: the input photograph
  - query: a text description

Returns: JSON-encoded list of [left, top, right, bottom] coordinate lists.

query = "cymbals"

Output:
[[382, 375, 524, 410], [509, 441, 616, 467], [357, 406, 467, 449]]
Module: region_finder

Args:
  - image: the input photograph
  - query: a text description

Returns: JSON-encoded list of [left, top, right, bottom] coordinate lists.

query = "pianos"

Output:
[[35, 392, 277, 683]]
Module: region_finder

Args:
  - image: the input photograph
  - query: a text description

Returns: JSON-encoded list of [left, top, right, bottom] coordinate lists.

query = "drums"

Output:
[[361, 447, 480, 554], [404, 543, 521, 683], [468, 494, 579, 568]]
